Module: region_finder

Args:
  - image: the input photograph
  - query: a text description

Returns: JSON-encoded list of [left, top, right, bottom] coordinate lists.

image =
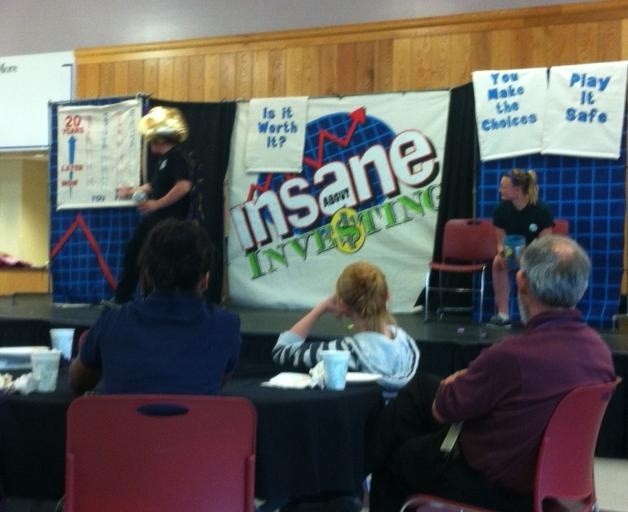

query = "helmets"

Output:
[[140, 106, 188, 143]]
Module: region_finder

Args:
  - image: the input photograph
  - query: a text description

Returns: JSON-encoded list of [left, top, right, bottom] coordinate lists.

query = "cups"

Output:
[[31, 351, 59, 393], [50, 328, 74, 360], [131, 190, 149, 214], [320, 350, 350, 392]]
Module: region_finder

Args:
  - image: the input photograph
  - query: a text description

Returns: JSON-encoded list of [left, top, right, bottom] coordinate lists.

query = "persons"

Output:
[[69, 220, 240, 396], [370, 235, 615, 512], [115, 107, 195, 305], [493, 170, 554, 326], [271, 260, 420, 406]]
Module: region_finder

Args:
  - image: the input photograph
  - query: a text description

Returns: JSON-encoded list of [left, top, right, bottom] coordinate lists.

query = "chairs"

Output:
[[422, 217, 569, 324], [56, 395, 268, 512], [400, 376, 624, 512]]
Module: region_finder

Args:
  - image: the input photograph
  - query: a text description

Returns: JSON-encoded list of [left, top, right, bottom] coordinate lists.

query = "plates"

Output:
[[346, 371, 382, 384], [0, 346, 49, 361]]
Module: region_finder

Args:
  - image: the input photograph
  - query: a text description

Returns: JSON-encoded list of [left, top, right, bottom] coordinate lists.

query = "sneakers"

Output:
[[486, 316, 511, 329]]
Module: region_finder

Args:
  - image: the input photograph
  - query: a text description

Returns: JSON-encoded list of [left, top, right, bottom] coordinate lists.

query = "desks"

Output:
[[0, 363, 380, 511]]
